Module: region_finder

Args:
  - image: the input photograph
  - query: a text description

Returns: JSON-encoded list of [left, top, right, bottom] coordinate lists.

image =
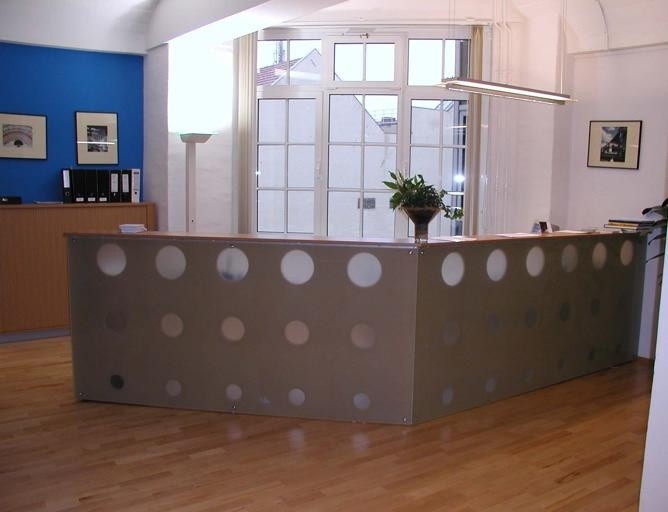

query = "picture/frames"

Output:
[[75, 112, 120, 166], [586, 118, 642, 169], [0, 113, 48, 161]]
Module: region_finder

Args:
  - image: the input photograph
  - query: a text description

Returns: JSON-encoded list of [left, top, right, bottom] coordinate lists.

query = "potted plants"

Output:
[[381, 166, 464, 236]]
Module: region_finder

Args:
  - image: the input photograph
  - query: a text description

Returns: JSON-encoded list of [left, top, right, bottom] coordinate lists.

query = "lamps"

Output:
[[443, 1, 575, 106]]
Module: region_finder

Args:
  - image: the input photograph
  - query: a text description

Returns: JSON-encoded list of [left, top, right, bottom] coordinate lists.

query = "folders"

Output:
[[62, 168, 141, 204]]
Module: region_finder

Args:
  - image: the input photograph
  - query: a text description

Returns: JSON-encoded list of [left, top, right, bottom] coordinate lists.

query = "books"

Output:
[[557, 229, 593, 234], [595, 219, 655, 233], [118, 224, 147, 233], [32, 200, 64, 204]]
